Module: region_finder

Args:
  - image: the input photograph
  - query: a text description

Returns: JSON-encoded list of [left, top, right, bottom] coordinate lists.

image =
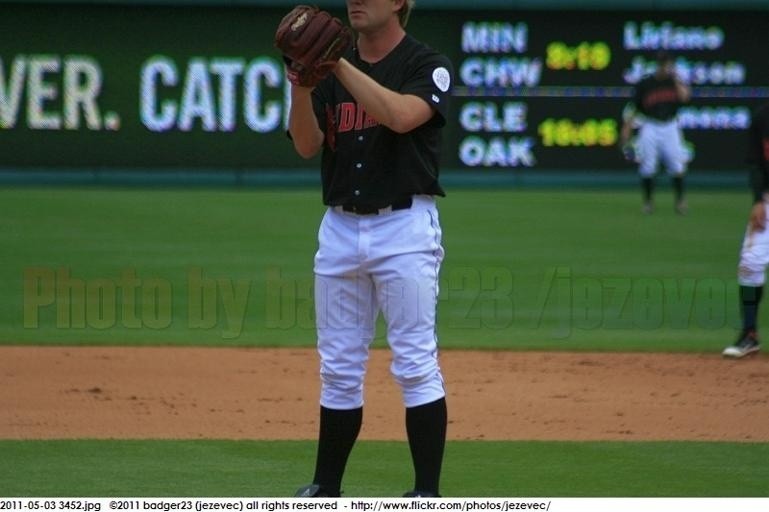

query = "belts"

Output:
[[342, 198, 412, 216]]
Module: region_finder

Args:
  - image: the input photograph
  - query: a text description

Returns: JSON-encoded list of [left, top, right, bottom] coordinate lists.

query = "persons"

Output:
[[721, 103, 768, 358], [619, 51, 693, 217], [277, 0, 453, 498]]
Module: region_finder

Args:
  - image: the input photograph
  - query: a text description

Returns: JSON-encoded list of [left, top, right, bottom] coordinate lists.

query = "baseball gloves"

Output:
[[275, 5, 357, 89]]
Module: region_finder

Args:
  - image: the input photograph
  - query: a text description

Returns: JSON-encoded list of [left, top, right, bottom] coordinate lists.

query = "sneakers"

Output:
[[722, 328, 761, 360]]
